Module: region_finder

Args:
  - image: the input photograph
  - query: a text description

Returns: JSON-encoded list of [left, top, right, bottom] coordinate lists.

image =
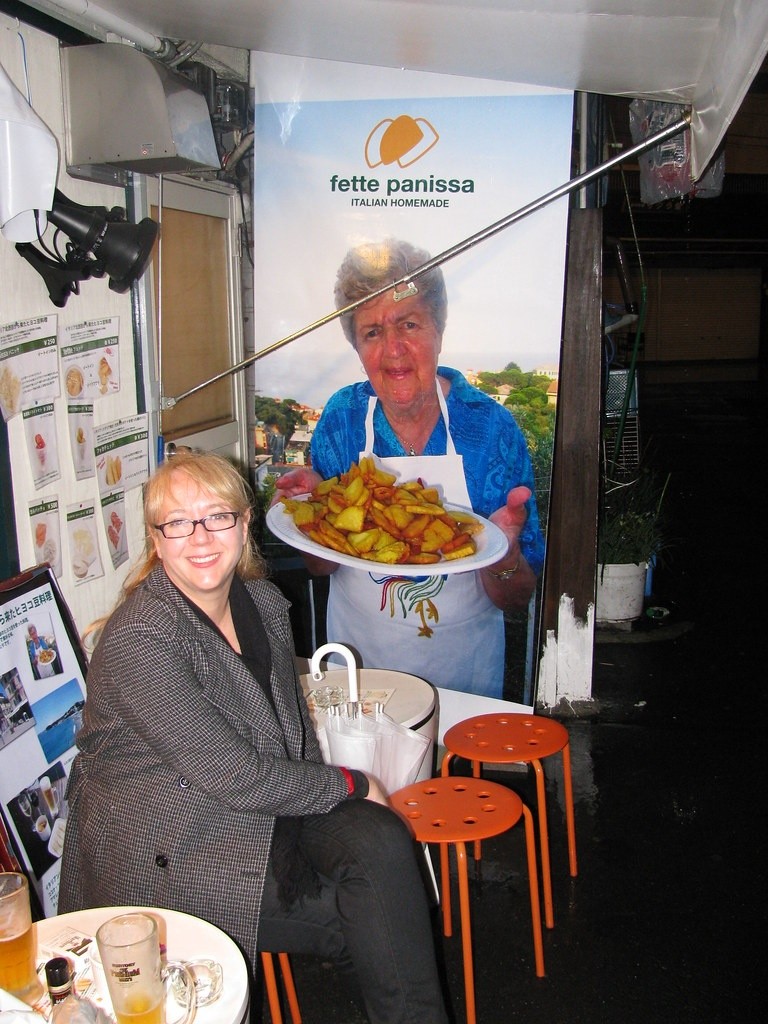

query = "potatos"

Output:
[[279, 455, 485, 564]]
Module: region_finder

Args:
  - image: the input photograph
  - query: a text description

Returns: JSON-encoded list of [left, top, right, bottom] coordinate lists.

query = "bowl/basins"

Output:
[[73, 560, 88, 578], [104, 472, 122, 490], [63, 363, 86, 399]]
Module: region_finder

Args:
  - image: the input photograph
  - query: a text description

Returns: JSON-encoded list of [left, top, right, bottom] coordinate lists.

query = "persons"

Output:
[[28, 623, 55, 679], [57, 454, 450, 1024], [265, 239, 543, 699]]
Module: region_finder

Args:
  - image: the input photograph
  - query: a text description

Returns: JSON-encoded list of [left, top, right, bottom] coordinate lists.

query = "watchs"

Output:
[[485, 561, 519, 582]]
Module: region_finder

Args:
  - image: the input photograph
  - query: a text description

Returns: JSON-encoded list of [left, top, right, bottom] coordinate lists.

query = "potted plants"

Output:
[[593, 505, 678, 623]]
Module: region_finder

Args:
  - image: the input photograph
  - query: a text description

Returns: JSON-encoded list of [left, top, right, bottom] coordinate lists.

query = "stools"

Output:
[[386, 776, 547, 1024], [437, 713, 579, 928]]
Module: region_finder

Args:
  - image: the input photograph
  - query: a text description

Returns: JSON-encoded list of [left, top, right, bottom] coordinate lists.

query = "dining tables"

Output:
[[30, 905, 251, 1024]]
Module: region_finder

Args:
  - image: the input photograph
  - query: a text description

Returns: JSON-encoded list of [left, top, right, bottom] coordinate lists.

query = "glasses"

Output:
[[154, 512, 242, 539]]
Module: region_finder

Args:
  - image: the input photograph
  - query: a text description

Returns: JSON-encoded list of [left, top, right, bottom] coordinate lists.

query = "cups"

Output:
[[96, 914, 197, 1024], [35, 444, 47, 470], [77, 441, 87, 468], [127, 911, 166, 962], [35, 814, 51, 841], [40, 776, 59, 817], [0, 871, 44, 1007], [89, 939, 110, 997]]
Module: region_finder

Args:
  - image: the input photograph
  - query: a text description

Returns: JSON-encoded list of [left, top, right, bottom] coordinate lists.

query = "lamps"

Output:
[[15, 188, 160, 308]]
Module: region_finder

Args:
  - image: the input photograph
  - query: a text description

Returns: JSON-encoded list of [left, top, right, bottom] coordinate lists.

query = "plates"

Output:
[[71, 521, 97, 566], [37, 649, 56, 665], [0, 988, 50, 1024], [266, 484, 509, 576], [47, 818, 67, 858]]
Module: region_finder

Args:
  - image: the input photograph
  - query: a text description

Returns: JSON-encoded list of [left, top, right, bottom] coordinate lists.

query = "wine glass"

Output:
[[27, 789, 42, 815], [18, 794, 37, 832]]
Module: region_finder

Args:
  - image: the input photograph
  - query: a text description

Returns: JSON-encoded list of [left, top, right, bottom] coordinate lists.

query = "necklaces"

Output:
[[395, 401, 438, 457]]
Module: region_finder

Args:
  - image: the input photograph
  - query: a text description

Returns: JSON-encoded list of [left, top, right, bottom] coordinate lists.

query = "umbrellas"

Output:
[[314, 643, 431, 798]]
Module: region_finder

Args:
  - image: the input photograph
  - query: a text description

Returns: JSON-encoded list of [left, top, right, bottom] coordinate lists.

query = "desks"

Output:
[[594, 618, 693, 647], [299, 669, 435, 782]]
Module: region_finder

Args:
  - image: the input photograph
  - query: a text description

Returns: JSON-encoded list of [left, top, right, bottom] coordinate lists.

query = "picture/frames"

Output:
[[0, 561, 89, 922]]
